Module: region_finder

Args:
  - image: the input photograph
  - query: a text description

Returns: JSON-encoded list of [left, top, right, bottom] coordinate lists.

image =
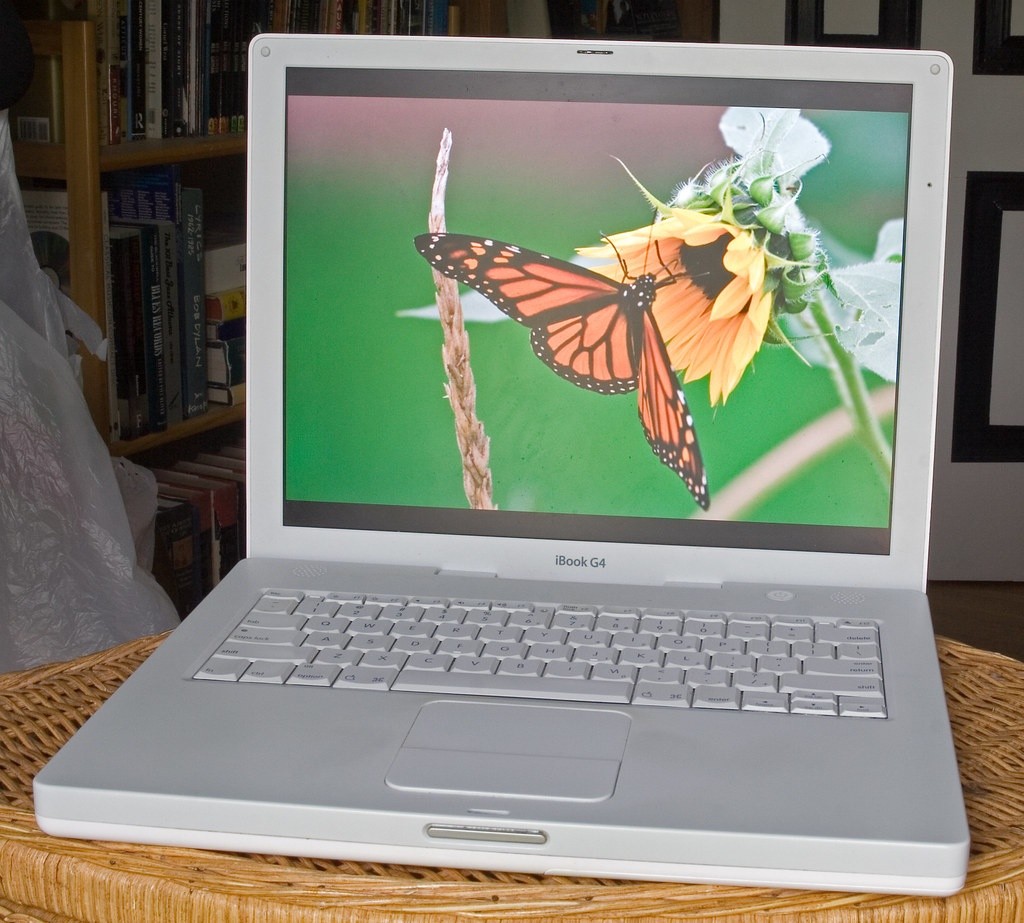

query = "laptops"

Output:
[[31, 32, 972, 896]]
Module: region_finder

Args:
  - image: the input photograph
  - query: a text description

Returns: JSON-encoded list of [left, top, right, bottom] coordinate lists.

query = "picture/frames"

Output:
[[786, 0, 1024, 463]]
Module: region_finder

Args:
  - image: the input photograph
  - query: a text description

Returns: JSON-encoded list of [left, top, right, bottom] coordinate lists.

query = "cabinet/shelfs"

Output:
[[0, 0, 509, 623]]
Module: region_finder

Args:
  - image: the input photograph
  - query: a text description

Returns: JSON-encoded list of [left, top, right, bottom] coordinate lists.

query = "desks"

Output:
[[0, 628, 1024, 923]]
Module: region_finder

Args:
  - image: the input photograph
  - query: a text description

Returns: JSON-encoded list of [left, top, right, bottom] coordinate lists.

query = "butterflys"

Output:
[[412, 207, 713, 512]]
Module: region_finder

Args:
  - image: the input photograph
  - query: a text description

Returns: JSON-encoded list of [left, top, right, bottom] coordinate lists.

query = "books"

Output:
[[147, 446, 247, 625], [22, 172, 246, 442], [0, 0, 459, 144]]
